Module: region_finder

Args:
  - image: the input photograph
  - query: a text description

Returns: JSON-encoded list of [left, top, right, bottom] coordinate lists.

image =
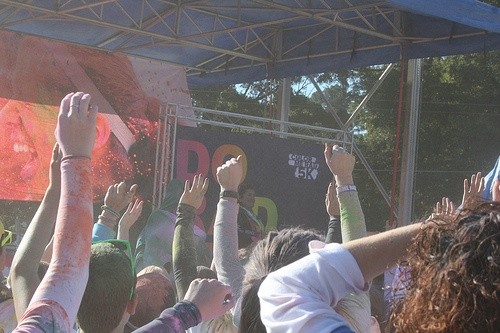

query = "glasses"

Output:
[[92, 239, 135, 300], [0, 230, 13, 246]]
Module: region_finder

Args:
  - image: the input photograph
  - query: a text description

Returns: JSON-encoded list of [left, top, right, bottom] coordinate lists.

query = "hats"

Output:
[[126, 266, 177, 331]]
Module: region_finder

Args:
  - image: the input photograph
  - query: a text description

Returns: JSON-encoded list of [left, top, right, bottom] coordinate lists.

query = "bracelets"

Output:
[[220, 191, 239, 199], [101, 205, 120, 218], [98, 215, 117, 224], [61, 154, 91, 160], [335, 184, 357, 194]]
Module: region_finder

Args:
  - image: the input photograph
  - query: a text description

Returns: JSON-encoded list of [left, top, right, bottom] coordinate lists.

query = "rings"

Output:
[[70, 105, 79, 107]]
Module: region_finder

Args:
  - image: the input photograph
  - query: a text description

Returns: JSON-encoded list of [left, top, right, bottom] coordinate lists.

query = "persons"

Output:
[[0, 91, 500, 333], [0, 33, 180, 204]]
